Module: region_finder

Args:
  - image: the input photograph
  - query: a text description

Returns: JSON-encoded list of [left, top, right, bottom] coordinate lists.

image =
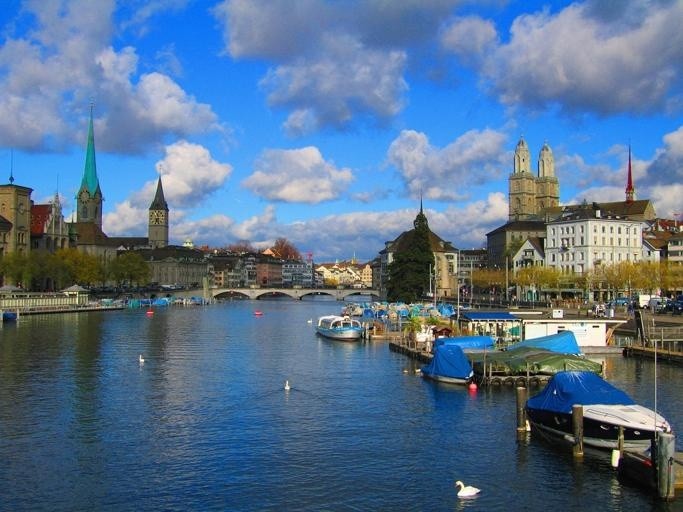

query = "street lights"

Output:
[[456, 245, 488, 301]]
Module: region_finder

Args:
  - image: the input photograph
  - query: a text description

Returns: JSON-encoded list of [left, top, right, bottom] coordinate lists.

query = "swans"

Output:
[[139, 355, 144, 363], [285, 380, 290, 390], [454, 480, 481, 497]]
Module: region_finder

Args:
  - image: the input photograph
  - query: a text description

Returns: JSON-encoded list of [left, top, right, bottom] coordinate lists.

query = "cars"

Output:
[[604, 297, 629, 306], [99, 281, 184, 294], [668, 295, 682, 312]]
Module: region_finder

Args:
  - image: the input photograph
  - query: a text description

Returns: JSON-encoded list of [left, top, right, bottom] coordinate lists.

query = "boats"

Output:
[[0, 312, 16, 320], [99, 295, 210, 307], [315, 315, 362, 342], [420, 345, 475, 386], [339, 301, 475, 321], [523, 371, 672, 459]]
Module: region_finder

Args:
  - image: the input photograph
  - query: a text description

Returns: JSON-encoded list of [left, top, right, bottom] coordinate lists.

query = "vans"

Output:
[[650, 297, 671, 310], [638, 294, 660, 309]]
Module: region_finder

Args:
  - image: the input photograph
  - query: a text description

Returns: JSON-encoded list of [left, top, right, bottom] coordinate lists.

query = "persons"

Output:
[[554, 297, 608, 318]]
